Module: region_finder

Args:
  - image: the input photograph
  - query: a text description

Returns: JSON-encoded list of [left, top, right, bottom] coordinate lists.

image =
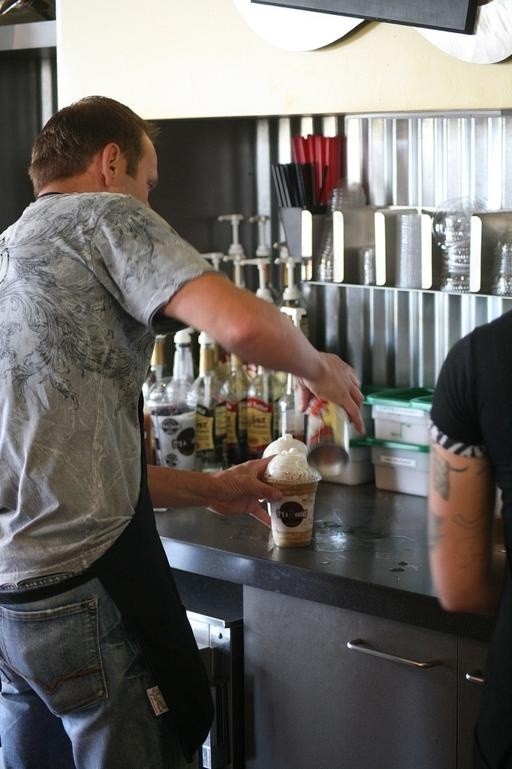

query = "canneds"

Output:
[[305, 397, 350, 479]]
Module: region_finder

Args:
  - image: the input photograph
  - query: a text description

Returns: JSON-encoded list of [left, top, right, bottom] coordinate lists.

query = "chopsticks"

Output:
[[269, 135, 343, 214]]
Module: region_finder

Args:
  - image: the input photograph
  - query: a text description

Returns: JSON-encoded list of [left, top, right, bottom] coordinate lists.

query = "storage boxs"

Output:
[[347, 386, 432, 500]]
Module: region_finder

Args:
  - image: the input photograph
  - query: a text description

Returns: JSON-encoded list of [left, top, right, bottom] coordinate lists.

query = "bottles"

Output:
[[142, 332, 349, 474]]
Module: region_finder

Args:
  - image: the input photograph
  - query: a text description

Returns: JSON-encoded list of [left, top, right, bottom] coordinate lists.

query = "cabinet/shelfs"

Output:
[[242, 585, 512, 769]]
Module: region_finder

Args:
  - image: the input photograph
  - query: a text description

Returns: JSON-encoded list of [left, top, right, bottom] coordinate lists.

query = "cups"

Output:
[[263, 474, 323, 548]]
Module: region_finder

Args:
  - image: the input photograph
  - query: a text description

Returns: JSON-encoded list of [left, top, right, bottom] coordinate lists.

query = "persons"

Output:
[[0, 95, 365, 769], [427, 310, 512, 768]]
